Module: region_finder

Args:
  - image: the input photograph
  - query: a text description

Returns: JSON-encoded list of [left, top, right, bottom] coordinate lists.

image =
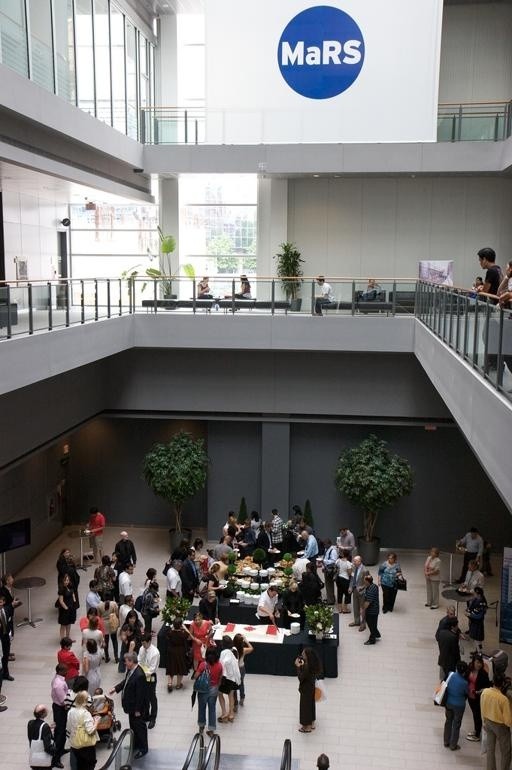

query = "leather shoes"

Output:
[[51, 760, 64, 768], [0, 653, 15, 712]]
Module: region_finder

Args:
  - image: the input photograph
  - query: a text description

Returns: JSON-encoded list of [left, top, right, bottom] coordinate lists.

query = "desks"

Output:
[[67, 530, 95, 572], [435, 540, 467, 588], [218, 586, 285, 606], [12, 577, 46, 628], [224, 574, 269, 582], [441, 589, 477, 620], [157, 606, 340, 678]]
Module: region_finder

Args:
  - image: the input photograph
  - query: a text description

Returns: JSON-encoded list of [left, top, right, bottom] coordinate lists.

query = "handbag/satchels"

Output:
[[434, 680, 448, 706], [395, 579, 407, 591], [110, 613, 119, 631], [464, 605, 487, 619], [30, 739, 56, 767], [163, 562, 172, 576], [73, 726, 97, 749], [134, 596, 146, 613]]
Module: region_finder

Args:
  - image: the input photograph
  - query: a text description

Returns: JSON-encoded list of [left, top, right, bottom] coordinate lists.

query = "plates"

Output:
[[235, 567, 283, 605], [315, 558, 323, 561], [274, 551, 280, 553], [296, 551, 304, 554], [290, 622, 301, 635], [232, 548, 240, 552], [290, 613, 300, 618]]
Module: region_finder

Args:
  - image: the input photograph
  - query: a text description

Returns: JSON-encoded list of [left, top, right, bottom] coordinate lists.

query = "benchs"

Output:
[[140, 298, 215, 315], [389, 290, 485, 315], [356, 288, 387, 314], [321, 299, 392, 316], [219, 299, 291, 314]]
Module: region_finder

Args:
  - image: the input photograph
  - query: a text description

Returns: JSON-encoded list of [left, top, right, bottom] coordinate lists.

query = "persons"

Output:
[[293, 647, 319, 735], [27, 665, 114, 770], [229, 275, 251, 311], [0, 574, 19, 713], [54, 505, 222, 692], [109, 632, 159, 758], [221, 509, 403, 645], [190, 631, 252, 736], [470, 246, 512, 394], [312, 276, 335, 316], [316, 753, 330, 770], [361, 279, 382, 302], [423, 527, 511, 770], [197, 277, 213, 313]]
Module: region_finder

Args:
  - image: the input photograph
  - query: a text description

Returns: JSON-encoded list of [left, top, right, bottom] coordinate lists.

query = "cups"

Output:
[[337, 536, 342, 545]]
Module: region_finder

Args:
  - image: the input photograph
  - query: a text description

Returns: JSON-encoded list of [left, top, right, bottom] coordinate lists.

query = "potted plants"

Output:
[[331, 433, 417, 566], [139, 428, 214, 554], [271, 242, 306, 312], [121, 225, 196, 310]]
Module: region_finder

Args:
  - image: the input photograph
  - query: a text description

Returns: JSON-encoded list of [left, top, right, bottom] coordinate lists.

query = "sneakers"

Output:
[[467, 732, 480, 742], [322, 598, 388, 645], [104, 656, 244, 758]]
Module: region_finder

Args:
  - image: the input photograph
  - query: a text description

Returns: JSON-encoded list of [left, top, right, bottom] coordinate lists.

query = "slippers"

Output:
[[299, 726, 315, 732]]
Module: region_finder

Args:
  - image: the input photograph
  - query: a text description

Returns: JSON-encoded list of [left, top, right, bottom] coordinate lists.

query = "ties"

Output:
[[353, 567, 358, 587]]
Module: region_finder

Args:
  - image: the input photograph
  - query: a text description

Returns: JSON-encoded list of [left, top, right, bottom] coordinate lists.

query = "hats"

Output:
[[60, 637, 76, 645]]
